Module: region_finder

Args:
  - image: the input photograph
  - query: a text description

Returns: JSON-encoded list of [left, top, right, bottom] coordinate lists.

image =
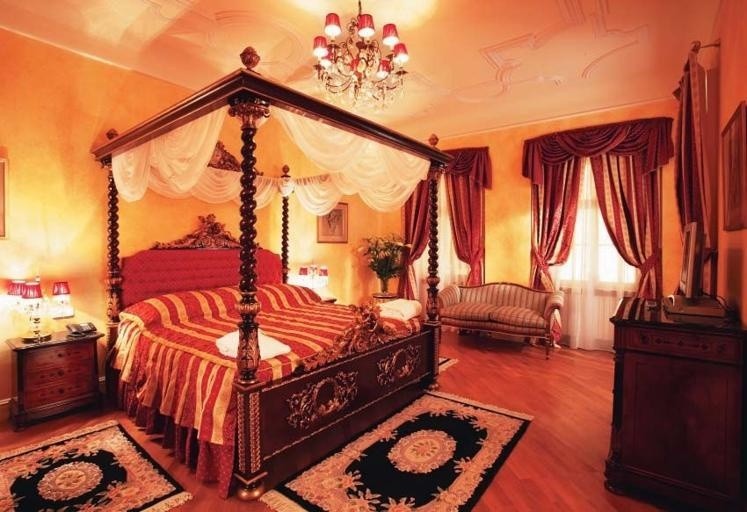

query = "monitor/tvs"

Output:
[[662, 222, 726, 319]]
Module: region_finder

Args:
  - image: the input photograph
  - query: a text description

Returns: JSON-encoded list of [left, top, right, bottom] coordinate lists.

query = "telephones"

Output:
[[66, 322, 96, 337]]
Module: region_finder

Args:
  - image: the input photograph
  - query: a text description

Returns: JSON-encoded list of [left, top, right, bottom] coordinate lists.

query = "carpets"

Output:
[[257, 387, 534, 512], [0, 417, 193, 512]]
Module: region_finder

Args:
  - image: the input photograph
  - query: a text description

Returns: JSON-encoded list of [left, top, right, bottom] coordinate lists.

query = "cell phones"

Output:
[[644, 297, 659, 310]]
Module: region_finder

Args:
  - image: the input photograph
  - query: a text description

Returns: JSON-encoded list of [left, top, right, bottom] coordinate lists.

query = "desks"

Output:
[[371, 292, 398, 301]]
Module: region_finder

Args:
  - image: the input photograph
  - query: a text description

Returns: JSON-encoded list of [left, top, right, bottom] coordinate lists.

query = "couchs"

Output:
[[434, 281, 565, 360]]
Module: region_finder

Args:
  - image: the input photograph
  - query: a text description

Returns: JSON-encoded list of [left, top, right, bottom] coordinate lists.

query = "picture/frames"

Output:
[[0, 158, 9, 241], [315, 202, 350, 246], [716, 99, 747, 232]]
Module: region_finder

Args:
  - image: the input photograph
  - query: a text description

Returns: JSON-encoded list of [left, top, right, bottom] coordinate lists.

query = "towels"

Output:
[[215, 326, 292, 361], [375, 298, 423, 322]]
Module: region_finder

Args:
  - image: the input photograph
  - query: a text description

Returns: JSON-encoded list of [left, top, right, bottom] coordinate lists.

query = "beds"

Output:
[[88, 44, 452, 501]]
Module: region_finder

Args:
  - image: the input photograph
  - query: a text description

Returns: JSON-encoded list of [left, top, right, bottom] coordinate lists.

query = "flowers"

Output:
[[354, 233, 411, 280]]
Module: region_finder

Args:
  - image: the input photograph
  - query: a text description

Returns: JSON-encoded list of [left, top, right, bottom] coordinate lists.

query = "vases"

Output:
[[377, 276, 392, 292]]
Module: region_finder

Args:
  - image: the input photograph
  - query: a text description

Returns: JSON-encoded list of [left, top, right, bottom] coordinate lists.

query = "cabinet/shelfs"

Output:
[[603, 296, 746, 512]]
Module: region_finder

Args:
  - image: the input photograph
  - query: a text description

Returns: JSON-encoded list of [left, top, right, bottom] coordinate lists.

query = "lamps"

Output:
[[7, 279, 53, 345], [312, 0, 411, 109], [294, 264, 329, 291], [52, 282, 75, 320]]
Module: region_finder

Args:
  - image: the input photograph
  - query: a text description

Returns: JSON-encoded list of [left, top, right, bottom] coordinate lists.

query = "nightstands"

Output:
[[5, 330, 105, 433], [321, 295, 337, 302]]
